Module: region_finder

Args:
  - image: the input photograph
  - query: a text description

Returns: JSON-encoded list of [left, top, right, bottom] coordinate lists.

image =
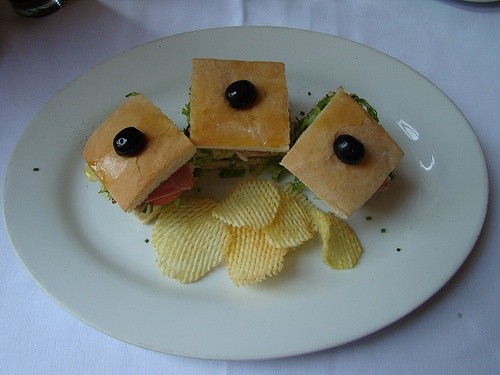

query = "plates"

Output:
[[1, 26, 489, 361]]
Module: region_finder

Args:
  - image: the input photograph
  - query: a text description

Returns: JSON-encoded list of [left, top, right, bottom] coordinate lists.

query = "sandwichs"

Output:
[[81, 93, 198, 224], [277, 86, 404, 221], [186, 57, 292, 174]]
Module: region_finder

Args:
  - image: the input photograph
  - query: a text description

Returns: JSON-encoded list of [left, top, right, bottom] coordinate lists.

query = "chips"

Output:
[[150, 178, 362, 290]]
